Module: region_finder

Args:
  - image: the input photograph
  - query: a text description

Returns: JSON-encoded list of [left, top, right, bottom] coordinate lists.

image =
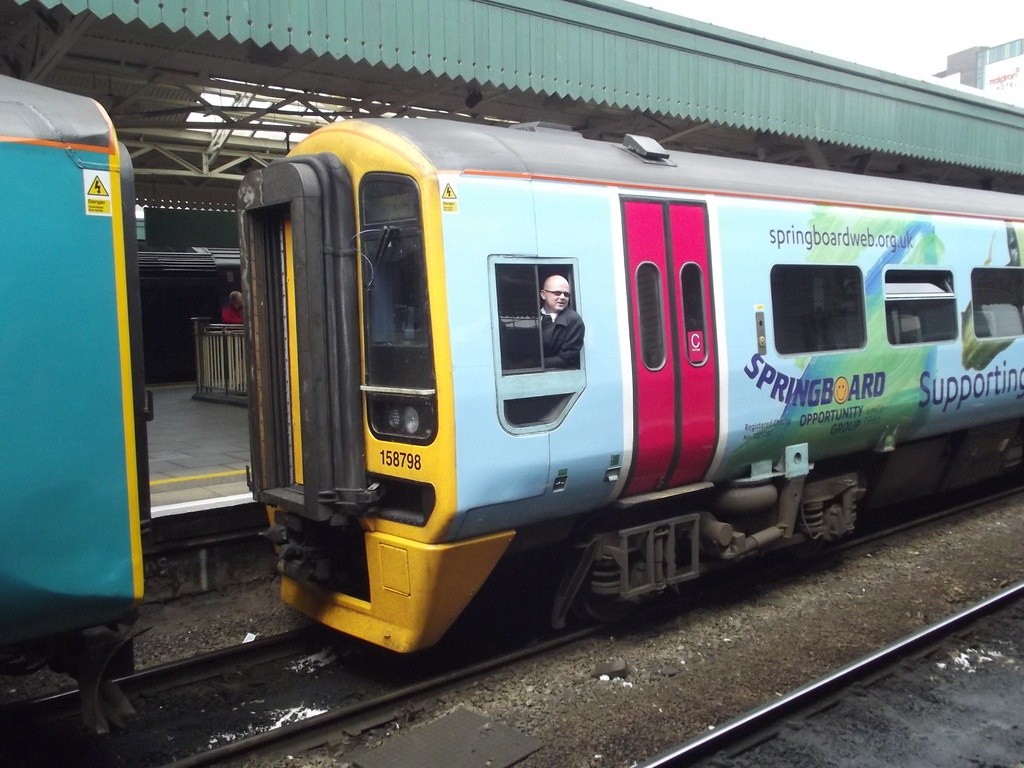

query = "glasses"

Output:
[[545, 289, 571, 297]]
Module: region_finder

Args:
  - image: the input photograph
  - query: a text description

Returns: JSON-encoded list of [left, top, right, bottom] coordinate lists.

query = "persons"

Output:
[[222, 289, 245, 391], [520, 274, 586, 368]]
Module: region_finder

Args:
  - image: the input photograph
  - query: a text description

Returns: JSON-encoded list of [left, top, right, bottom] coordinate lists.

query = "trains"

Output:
[[0, 71, 1023, 748]]
